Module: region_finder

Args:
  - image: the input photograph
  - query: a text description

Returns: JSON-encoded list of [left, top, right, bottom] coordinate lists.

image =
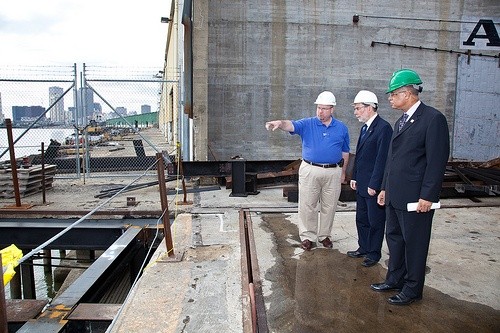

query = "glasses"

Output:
[[391, 91, 406, 96], [317, 107, 332, 111], [353, 107, 360, 110]]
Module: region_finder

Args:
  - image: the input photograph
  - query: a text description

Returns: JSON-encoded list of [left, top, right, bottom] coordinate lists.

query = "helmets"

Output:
[[354, 90, 379, 104], [385, 68, 422, 94], [314, 91, 336, 106]]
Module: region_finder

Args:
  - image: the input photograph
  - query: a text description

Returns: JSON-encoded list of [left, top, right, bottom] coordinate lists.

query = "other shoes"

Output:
[[302, 239, 312, 251], [361, 258, 376, 266], [347, 250, 367, 257], [319, 238, 333, 248]]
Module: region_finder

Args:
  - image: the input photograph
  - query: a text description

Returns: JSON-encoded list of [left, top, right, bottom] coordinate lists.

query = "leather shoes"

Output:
[[387, 291, 416, 304], [370, 282, 401, 291]]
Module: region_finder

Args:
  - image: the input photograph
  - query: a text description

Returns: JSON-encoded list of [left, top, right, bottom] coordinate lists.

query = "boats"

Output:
[[27, 123, 41, 129], [65, 119, 140, 145]]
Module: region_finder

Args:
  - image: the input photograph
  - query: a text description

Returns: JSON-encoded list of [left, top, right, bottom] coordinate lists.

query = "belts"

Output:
[[303, 159, 336, 168]]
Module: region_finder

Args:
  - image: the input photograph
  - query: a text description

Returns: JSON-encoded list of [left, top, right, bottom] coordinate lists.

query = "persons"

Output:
[[265, 93, 350, 250], [369, 73, 450, 305], [346, 93, 393, 267]]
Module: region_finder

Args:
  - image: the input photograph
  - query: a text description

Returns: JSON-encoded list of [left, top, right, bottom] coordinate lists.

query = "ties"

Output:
[[398, 114, 408, 133], [360, 124, 367, 142]]
